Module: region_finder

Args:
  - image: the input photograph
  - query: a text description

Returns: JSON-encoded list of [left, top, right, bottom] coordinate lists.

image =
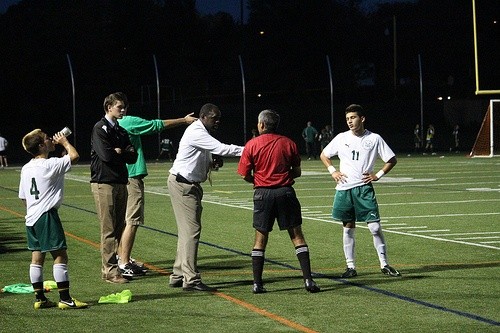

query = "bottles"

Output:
[[50, 126, 72, 144]]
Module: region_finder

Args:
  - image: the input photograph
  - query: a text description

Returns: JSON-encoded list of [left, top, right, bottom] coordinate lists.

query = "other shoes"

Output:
[[106, 274, 129, 284]]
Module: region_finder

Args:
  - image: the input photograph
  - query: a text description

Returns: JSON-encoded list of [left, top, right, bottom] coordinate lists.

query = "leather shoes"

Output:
[[169, 280, 184, 288], [183, 281, 217, 292]]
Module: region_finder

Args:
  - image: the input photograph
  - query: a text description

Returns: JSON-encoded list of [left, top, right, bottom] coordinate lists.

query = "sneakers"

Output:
[[382, 264, 401, 277], [253, 283, 267, 294], [304, 279, 320, 293], [59, 298, 89, 310], [341, 269, 357, 278], [115, 255, 149, 276], [34, 298, 56, 309]]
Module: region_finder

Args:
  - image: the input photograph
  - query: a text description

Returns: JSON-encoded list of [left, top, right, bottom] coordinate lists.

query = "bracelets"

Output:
[[375, 170, 384, 178], [328, 166, 336, 173]]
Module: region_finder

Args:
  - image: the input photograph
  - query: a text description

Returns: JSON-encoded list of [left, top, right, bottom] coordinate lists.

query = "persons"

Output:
[[115, 92, 199, 276], [0, 137, 8, 167], [19, 129, 88, 309], [167, 104, 243, 290], [90, 92, 138, 283], [156, 135, 174, 161], [320, 105, 400, 278], [251, 129, 257, 139], [423, 124, 436, 154], [302, 122, 331, 160], [414, 124, 422, 155], [453, 125, 461, 150], [238, 110, 320, 293]]
